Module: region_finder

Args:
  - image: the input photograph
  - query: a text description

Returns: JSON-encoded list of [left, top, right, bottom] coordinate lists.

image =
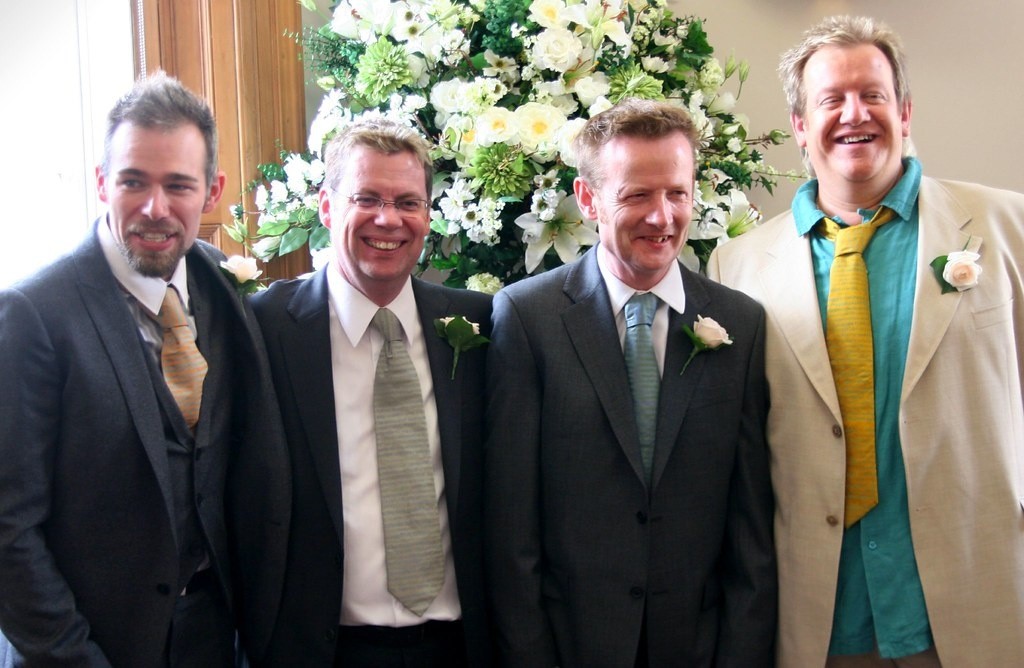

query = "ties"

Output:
[[816, 206, 894, 531], [620, 292, 663, 488], [367, 309, 446, 617], [142, 287, 209, 428]]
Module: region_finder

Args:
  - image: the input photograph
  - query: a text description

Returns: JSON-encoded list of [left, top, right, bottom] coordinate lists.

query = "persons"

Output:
[[484, 100, 779, 668], [0, 66, 290, 667], [258, 135, 494, 668], [707, 9, 1024, 668]]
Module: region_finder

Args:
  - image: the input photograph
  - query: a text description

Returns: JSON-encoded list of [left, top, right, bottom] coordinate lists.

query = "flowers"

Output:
[[678, 314, 735, 376], [221, 0, 814, 295], [218, 256, 272, 319], [930, 250, 983, 295], [433, 315, 492, 380]]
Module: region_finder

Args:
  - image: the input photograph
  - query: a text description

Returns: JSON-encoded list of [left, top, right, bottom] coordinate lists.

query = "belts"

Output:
[[179, 566, 214, 597]]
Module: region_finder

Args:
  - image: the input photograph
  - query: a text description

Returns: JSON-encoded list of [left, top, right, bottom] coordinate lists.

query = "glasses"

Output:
[[326, 184, 431, 221]]
